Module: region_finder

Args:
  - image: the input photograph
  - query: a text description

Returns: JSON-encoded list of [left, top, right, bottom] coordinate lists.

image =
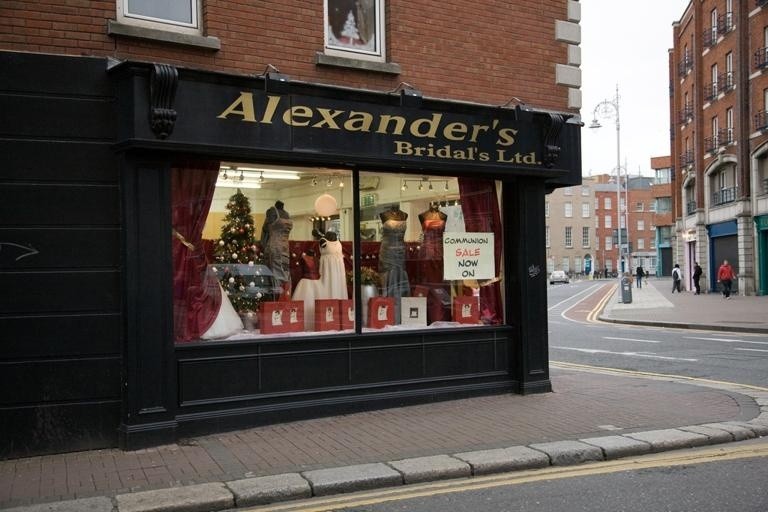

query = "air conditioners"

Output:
[[254, 60, 290, 97]]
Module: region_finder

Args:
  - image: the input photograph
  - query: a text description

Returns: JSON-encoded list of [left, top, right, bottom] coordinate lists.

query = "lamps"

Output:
[[499, 95, 533, 122], [222, 168, 264, 184], [400, 178, 449, 192], [384, 80, 424, 108]]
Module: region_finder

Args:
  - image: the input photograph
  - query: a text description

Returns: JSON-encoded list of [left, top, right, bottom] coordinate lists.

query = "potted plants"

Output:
[[347, 265, 382, 327]]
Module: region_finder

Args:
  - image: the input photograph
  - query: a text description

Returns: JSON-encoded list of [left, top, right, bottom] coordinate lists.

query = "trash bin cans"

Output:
[[607, 272, 611, 278], [621, 277, 632, 304], [612, 272, 618, 277]]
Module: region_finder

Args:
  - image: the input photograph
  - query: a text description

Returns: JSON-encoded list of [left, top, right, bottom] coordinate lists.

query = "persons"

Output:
[[643, 270, 650, 287], [715, 259, 737, 300], [635, 264, 645, 288], [258, 200, 292, 302], [317, 230, 349, 301], [416, 201, 447, 324], [690, 261, 702, 296], [669, 263, 682, 294], [378, 204, 413, 326]]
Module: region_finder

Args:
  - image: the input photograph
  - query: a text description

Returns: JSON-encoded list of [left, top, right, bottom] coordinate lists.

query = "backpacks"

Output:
[[673, 271, 679, 281]]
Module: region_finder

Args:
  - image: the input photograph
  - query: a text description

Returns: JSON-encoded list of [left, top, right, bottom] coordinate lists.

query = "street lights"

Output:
[[588, 81, 623, 304], [606, 155, 631, 275]]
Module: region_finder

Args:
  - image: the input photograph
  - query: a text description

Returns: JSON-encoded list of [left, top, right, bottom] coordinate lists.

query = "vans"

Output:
[[209, 263, 281, 302]]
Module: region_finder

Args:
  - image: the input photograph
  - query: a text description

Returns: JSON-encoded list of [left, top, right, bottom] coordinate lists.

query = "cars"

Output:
[[549, 270, 570, 285]]
